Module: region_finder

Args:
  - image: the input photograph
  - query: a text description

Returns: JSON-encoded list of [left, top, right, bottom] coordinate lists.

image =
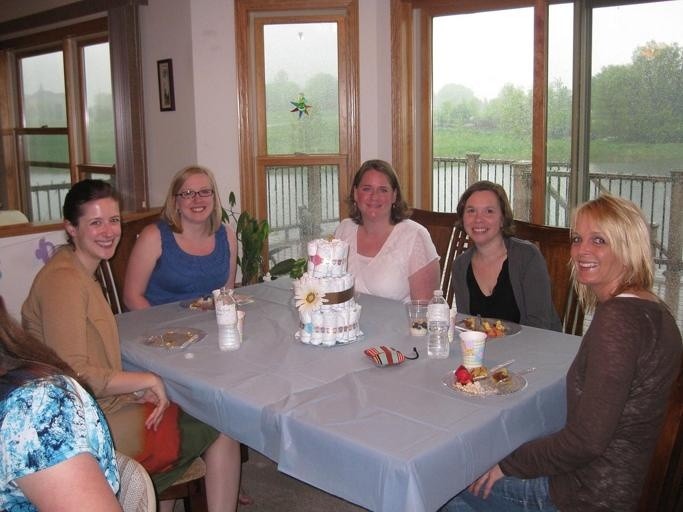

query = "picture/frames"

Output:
[[155, 58, 175, 113]]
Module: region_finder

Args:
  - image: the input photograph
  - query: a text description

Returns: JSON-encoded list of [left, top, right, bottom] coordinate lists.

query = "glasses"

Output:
[[175, 190, 213, 199]]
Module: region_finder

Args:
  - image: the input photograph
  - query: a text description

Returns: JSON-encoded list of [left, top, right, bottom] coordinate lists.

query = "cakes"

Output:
[[291, 235, 365, 347]]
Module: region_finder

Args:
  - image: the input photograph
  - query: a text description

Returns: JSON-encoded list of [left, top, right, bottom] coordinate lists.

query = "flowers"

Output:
[[293, 277, 329, 316]]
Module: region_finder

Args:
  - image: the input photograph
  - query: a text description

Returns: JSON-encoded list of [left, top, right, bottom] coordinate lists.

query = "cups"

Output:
[[405, 299, 428, 337], [211, 289, 234, 305], [235, 310, 245, 344], [446, 309, 457, 343], [458, 331, 487, 369]]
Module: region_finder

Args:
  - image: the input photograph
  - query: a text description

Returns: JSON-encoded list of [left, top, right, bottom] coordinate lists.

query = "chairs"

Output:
[[402, 204, 588, 335], [625, 371, 683, 511]]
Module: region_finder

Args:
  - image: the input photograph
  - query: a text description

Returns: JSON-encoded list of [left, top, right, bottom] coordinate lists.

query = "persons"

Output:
[[0, 295, 126, 512], [447, 179, 564, 334], [436, 194, 683, 511], [121, 166, 240, 313], [26, 178, 242, 511], [328, 158, 441, 307]]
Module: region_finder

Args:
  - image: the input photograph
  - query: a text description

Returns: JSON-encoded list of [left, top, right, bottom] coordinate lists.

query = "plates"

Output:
[[135, 326, 209, 353], [441, 366, 528, 399], [455, 318, 522, 341]]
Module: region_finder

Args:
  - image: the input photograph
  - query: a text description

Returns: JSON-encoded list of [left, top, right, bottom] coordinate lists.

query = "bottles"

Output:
[[425, 290, 450, 360], [214, 286, 241, 352]]
